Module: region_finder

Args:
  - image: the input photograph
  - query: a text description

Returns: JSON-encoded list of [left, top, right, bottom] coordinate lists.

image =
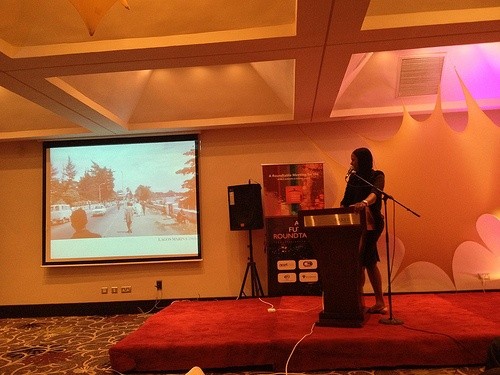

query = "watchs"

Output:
[[362, 200, 368, 206]]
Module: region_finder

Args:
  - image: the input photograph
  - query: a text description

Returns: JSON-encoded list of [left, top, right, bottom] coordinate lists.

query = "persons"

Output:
[[70, 208, 101, 238], [115, 200, 146, 232], [340, 147, 387, 314]]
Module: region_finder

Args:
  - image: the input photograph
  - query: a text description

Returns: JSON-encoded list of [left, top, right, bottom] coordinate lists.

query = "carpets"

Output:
[[109, 292, 499, 372]]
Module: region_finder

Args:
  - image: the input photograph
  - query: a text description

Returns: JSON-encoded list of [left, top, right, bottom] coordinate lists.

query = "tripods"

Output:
[[236, 230, 265, 301]]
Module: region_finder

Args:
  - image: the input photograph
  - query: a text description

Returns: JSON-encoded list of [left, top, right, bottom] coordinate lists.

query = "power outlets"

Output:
[[121, 286, 131, 293], [111, 287, 117, 293], [101, 287, 108, 294]]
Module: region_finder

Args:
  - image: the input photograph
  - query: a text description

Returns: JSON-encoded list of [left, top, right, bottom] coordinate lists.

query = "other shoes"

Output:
[[367, 304, 387, 315]]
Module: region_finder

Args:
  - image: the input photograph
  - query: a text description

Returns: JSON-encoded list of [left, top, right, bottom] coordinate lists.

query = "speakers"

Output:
[[228, 184, 264, 230]]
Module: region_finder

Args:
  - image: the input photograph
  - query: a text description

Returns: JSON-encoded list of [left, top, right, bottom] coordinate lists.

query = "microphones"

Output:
[[345, 166, 354, 179]]
